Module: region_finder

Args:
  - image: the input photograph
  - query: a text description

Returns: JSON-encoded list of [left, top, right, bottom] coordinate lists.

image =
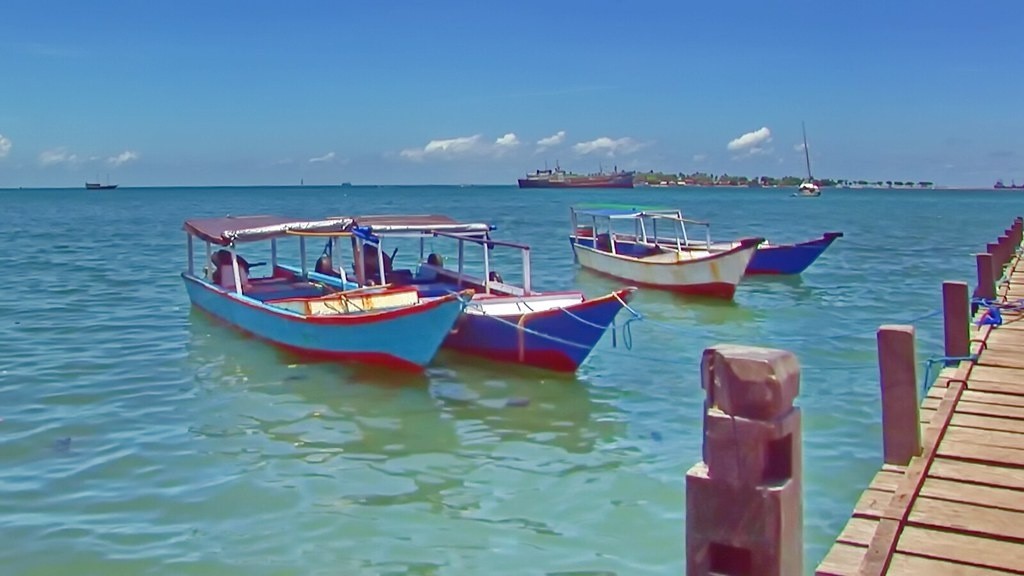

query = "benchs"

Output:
[[239, 282, 322, 302]]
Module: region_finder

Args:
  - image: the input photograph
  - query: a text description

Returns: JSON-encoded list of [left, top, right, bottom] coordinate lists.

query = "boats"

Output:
[[86, 182, 118, 190], [326, 213, 639, 374], [518, 160, 636, 188], [181, 214, 476, 372], [569, 223, 844, 276], [570, 203, 766, 301]]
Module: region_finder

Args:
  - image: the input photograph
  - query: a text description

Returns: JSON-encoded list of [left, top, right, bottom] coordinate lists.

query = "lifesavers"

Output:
[[489, 271, 503, 283]]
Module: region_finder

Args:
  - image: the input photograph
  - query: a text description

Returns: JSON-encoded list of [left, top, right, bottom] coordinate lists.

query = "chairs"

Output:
[[385, 269, 413, 288], [595, 234, 616, 252]]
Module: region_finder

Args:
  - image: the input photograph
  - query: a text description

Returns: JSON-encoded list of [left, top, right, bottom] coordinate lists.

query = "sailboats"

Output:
[[799, 121, 821, 196]]
